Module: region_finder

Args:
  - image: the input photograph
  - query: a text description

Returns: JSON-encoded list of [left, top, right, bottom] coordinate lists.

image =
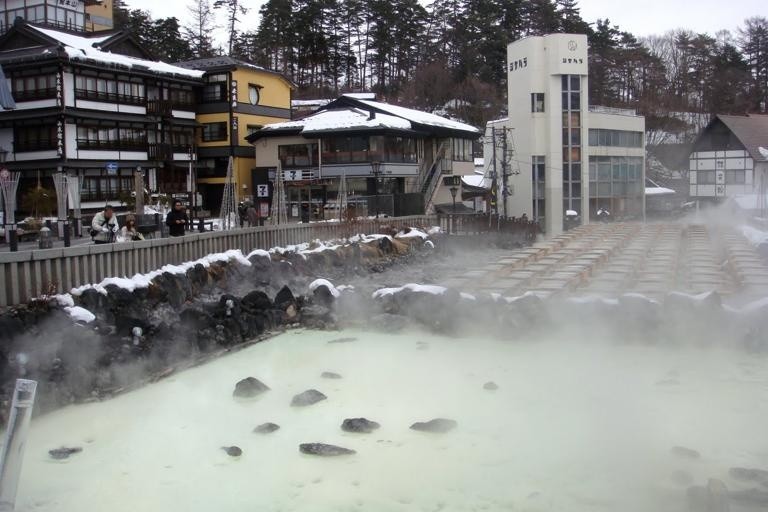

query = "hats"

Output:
[[125, 215, 136, 222]]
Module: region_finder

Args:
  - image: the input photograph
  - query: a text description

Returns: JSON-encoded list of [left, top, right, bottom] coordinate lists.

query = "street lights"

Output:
[[369, 159, 383, 218], [450, 186, 458, 233]]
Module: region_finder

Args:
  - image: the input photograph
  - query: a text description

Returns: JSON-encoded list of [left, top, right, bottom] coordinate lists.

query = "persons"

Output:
[[120, 215, 138, 241], [91, 205, 119, 245], [165, 199, 189, 238], [238, 201, 245, 228], [246, 205, 257, 227]]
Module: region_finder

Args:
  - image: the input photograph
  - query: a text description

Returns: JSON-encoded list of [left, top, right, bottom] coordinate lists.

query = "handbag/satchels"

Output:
[[88, 228, 99, 237], [116, 233, 145, 242]]
